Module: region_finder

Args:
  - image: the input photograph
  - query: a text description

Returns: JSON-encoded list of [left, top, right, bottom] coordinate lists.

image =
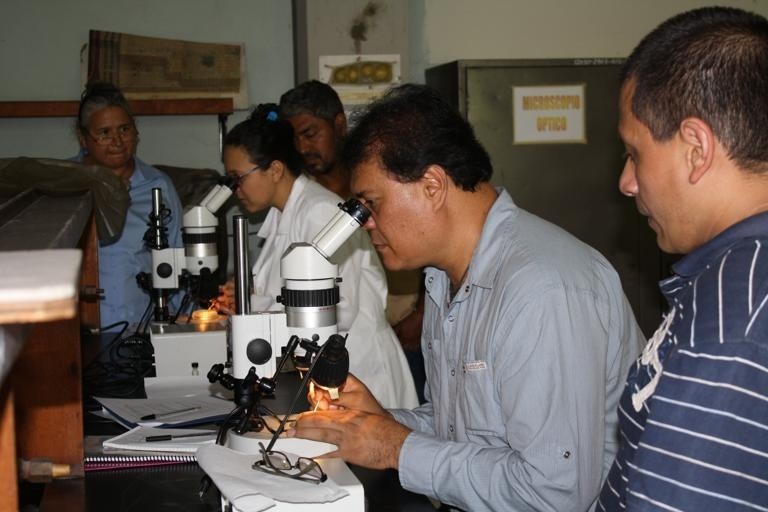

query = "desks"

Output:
[[0, 186, 439, 512]]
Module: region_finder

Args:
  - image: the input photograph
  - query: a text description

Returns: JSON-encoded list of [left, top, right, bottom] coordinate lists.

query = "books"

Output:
[[83, 425, 219, 470]]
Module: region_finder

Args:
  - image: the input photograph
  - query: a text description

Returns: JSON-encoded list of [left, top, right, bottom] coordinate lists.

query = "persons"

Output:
[[65, 80, 201, 333], [286, 83, 648, 512], [208, 103, 421, 410], [594, 7, 768, 512], [279, 80, 424, 404]]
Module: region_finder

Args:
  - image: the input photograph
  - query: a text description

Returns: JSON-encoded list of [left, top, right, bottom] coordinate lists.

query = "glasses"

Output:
[[250, 441, 327, 484], [85, 129, 136, 147], [235, 165, 263, 185]]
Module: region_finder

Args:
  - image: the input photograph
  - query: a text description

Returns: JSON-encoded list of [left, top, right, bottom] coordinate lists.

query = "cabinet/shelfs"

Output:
[[425, 56, 659, 341]]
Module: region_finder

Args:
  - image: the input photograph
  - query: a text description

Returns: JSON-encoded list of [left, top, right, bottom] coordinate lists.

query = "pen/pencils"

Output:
[[146, 432, 218, 442], [194, 475, 211, 500], [141, 406, 202, 421]]
